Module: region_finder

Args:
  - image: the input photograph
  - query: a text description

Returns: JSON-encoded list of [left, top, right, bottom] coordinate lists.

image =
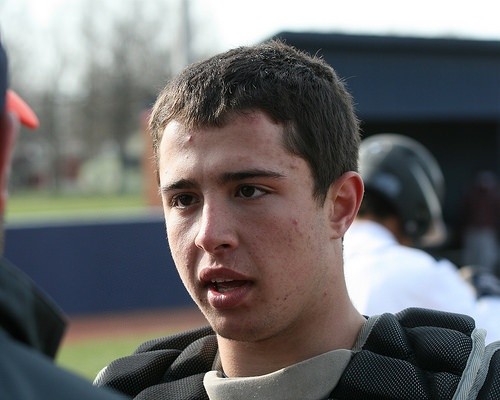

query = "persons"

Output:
[[93, 44, 500, 400], [343, 133, 500, 347]]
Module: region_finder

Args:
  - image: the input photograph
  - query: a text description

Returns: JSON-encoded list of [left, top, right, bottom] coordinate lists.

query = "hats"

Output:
[[0, 43, 40, 127]]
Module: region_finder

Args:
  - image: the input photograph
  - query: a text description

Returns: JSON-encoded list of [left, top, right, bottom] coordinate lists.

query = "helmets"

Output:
[[357, 134, 447, 246]]
